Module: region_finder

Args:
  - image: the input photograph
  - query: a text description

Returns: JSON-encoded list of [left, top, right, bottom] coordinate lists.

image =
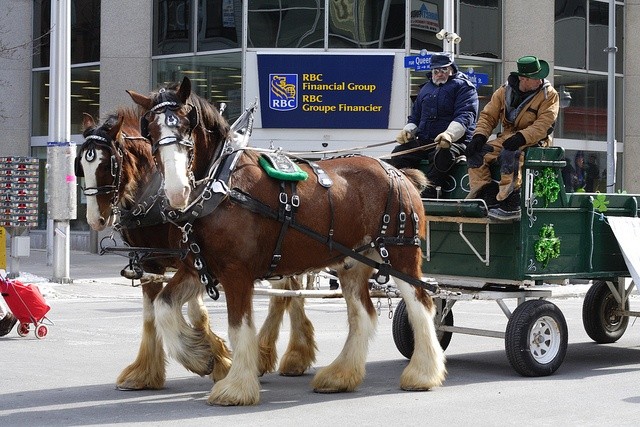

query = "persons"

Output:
[[390, 50, 479, 198], [464, 54, 559, 220]]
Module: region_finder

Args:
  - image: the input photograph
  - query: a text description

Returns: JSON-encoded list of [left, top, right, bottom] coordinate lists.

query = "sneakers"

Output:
[[0, 312, 17, 336]]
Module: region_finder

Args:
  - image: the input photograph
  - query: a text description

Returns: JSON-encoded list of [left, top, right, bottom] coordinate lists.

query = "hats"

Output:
[[510, 56, 549, 79], [430, 55, 458, 74]]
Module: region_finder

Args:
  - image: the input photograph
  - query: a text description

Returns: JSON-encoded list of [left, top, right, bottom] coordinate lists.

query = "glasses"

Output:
[[431, 68, 449, 74]]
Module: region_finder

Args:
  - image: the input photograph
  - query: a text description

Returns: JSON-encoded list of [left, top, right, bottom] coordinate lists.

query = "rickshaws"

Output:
[[4, 259, 51, 341]]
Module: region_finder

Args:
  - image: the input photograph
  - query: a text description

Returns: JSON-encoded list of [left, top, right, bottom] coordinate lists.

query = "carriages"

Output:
[[75, 77, 640, 406]]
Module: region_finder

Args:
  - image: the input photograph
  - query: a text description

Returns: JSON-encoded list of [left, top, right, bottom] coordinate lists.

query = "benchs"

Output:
[[382, 145, 568, 217]]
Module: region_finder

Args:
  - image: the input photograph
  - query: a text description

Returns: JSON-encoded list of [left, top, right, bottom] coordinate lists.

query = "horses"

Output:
[[74, 103, 320, 392], [123, 74, 449, 406]]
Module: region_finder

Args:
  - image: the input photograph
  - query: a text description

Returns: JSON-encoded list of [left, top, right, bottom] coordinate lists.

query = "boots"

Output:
[[478, 181, 500, 210], [488, 193, 522, 222]]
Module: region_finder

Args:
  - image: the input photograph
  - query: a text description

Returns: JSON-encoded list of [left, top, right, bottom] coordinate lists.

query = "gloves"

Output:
[[434, 121, 465, 150], [465, 134, 488, 157], [396, 123, 417, 144], [502, 132, 526, 151]]
